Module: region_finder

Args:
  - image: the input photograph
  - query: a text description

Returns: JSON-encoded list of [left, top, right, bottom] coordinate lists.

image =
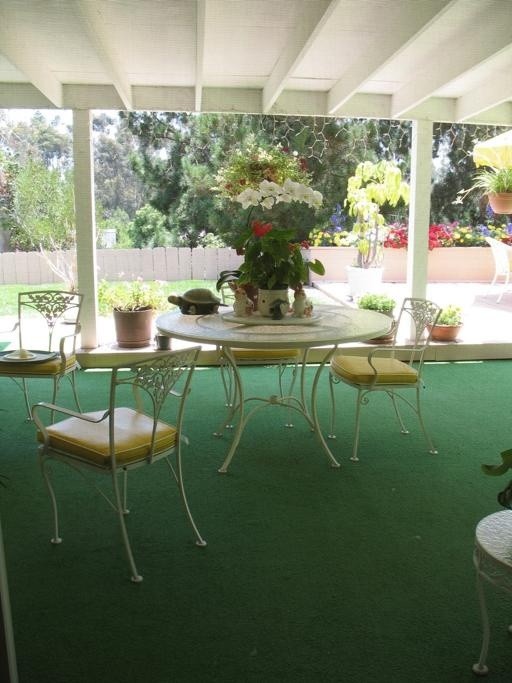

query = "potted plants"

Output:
[[461, 149, 512, 218], [330, 156, 412, 298], [354, 294, 397, 346], [94, 270, 178, 350], [424, 304, 466, 343]]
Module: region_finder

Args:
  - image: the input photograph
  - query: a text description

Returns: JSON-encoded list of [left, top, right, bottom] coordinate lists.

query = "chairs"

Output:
[[1, 286, 88, 430], [326, 293, 444, 464], [27, 339, 210, 583], [481, 234, 512, 305], [214, 273, 307, 430]]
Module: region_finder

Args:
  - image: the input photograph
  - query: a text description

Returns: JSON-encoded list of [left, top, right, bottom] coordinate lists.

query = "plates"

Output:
[[3, 352, 36, 360], [223, 310, 324, 326]]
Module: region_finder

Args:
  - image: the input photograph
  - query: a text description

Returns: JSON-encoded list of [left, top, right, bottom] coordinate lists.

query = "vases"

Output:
[[254, 287, 292, 318]]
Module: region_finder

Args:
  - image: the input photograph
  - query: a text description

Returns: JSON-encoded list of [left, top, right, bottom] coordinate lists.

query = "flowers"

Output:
[[206, 145, 326, 291]]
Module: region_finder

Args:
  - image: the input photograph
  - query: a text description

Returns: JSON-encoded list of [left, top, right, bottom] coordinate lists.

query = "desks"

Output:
[[153, 302, 394, 474]]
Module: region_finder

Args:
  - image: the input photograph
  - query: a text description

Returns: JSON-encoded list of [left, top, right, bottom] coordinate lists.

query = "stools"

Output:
[[466, 508, 512, 681]]
